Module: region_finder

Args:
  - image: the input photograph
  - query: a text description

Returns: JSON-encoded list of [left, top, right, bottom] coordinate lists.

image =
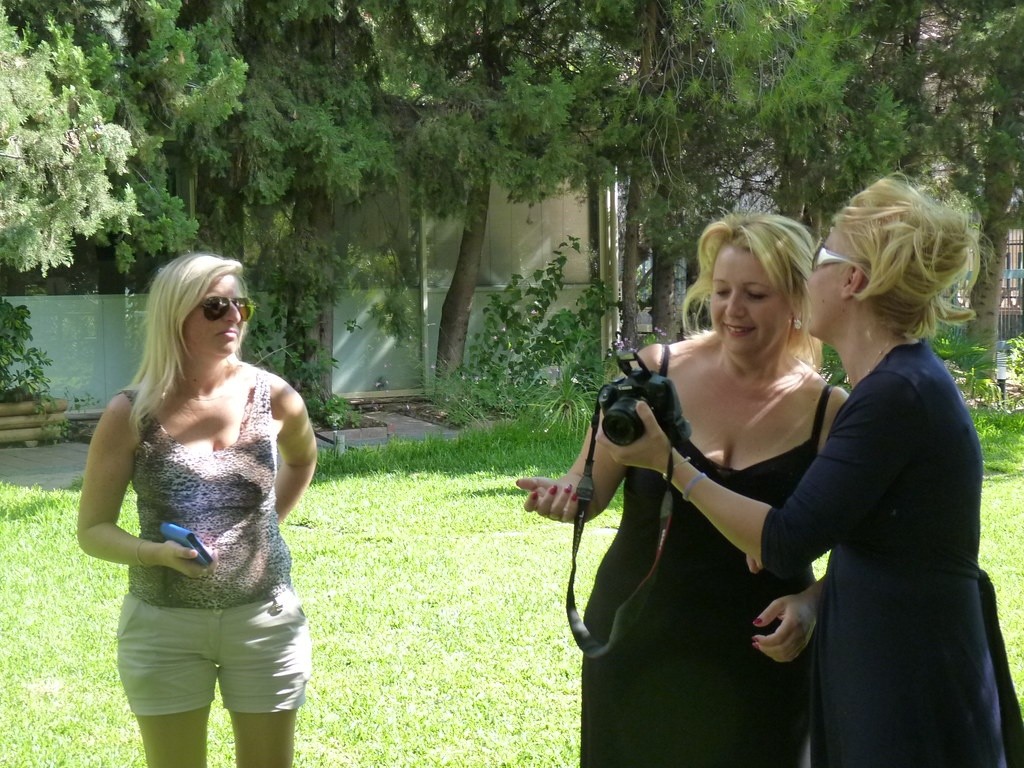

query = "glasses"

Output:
[[816, 244, 868, 267], [198, 295, 257, 322]]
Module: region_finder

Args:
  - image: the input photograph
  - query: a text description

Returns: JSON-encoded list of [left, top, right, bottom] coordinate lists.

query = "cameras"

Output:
[[599, 351, 692, 447]]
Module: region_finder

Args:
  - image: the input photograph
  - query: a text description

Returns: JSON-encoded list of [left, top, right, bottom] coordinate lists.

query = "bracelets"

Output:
[[662, 456, 692, 481], [683, 473, 708, 500], [136, 540, 153, 567]]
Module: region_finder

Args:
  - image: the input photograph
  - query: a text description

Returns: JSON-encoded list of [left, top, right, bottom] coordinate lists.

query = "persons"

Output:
[[595, 179, 1024, 768], [77, 255, 319, 768], [516, 212, 852, 767]]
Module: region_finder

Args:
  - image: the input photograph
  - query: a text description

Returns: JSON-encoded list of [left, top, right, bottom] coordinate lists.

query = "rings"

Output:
[[549, 514, 560, 521]]
